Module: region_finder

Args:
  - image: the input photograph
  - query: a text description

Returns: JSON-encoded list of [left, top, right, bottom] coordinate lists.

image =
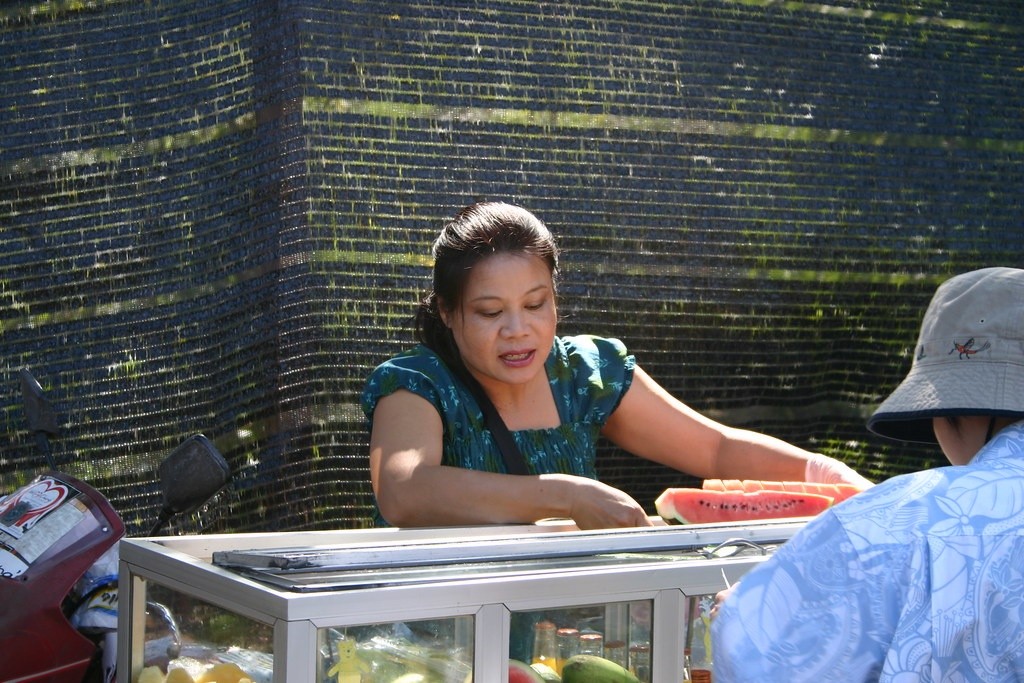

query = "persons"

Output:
[[709, 267, 1024, 683], [358, 200, 876, 666]]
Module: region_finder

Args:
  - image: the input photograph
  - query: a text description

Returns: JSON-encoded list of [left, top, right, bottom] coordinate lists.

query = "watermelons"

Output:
[[655, 480, 861, 525], [509, 655, 643, 683]]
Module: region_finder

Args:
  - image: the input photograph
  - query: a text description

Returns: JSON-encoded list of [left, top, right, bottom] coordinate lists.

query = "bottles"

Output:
[[604, 641, 624, 668], [555, 629, 580, 677], [684, 648, 692, 683], [579, 634, 603, 660], [533, 623, 557, 671], [629, 646, 649, 683]]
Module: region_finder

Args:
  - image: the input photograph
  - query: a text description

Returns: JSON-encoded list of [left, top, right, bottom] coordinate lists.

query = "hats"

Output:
[[866, 266, 1024, 444]]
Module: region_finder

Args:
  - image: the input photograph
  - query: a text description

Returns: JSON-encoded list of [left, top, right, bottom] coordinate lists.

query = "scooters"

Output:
[[0, 368, 232, 683]]
